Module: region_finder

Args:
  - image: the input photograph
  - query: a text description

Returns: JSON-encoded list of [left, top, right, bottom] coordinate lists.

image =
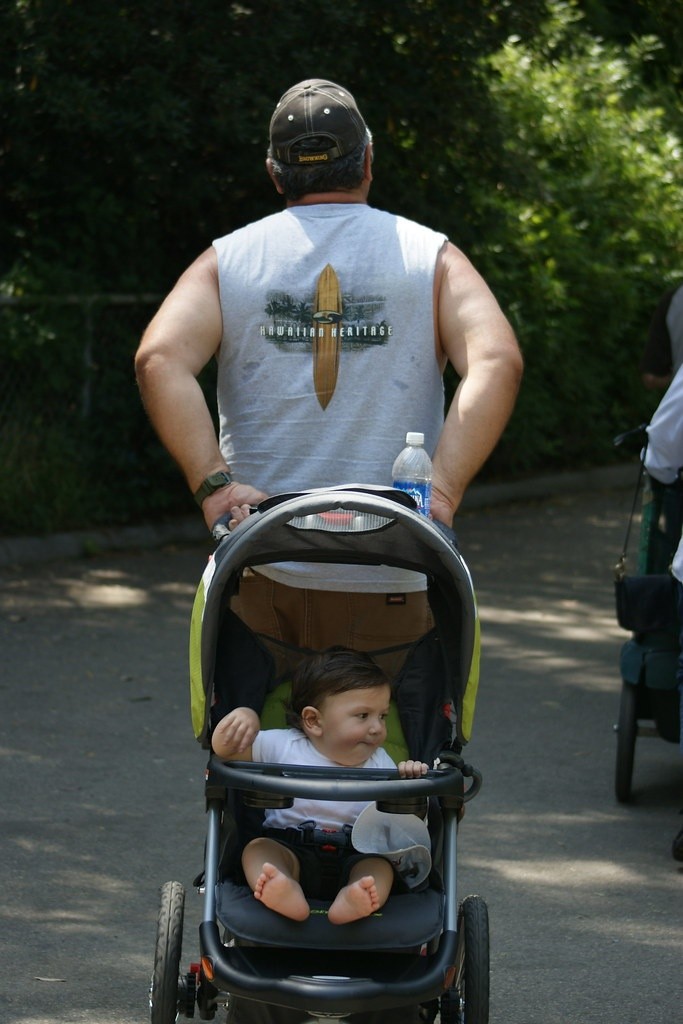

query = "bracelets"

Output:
[[194, 472, 233, 508]]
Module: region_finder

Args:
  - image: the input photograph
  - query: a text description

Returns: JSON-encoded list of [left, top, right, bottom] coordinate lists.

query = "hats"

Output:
[[352, 800, 432, 890], [270, 78, 367, 166]]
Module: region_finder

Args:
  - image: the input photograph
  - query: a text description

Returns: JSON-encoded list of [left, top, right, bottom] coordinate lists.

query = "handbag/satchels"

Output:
[[614, 573, 683, 633]]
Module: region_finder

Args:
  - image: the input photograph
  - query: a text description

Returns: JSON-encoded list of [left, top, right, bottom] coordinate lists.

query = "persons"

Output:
[[218, 644, 429, 925], [134, 77, 524, 678], [640, 365, 683, 862]]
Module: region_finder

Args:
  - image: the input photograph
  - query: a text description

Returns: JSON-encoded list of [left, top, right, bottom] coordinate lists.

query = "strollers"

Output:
[[148, 484, 491, 1024], [613, 423, 683, 805]]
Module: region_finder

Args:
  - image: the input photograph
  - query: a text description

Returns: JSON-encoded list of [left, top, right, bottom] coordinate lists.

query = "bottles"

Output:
[[392, 432, 432, 519]]
